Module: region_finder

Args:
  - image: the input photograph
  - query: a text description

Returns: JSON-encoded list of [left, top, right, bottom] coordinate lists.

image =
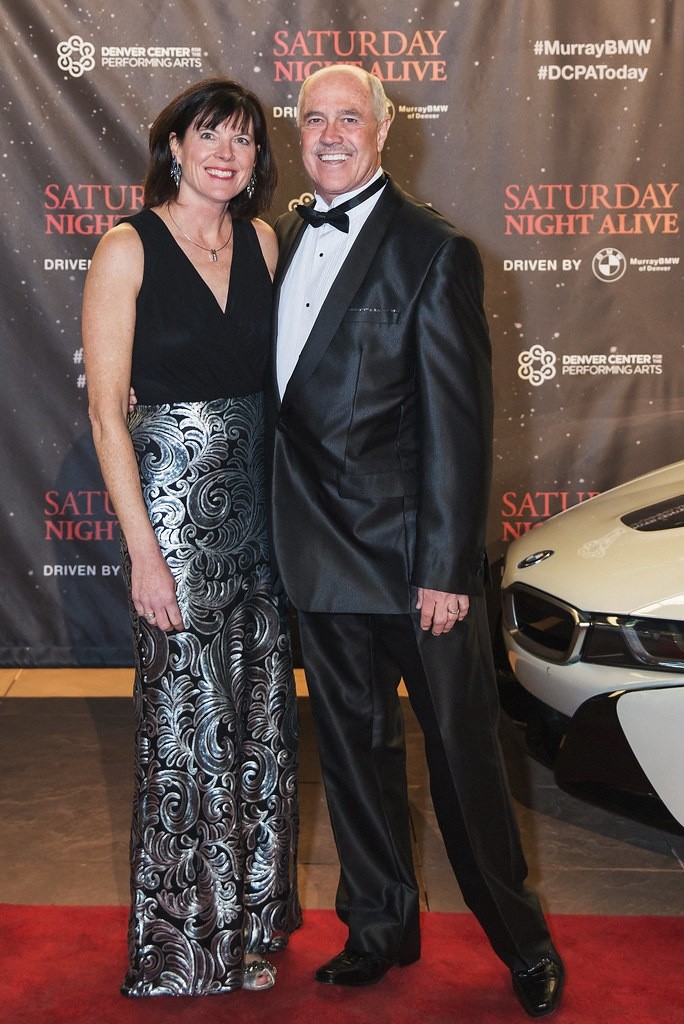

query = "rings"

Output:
[[143, 613, 154, 621], [448, 610, 459, 614]]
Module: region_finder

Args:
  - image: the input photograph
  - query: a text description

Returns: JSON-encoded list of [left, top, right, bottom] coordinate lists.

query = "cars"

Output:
[[489, 453, 684, 828]]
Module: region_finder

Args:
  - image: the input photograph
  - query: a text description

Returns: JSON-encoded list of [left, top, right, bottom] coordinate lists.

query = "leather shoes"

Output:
[[317, 938, 421, 985], [513, 946, 564, 1017]]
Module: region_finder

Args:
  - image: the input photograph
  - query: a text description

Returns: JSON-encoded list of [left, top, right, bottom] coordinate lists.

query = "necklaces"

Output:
[[167, 201, 234, 262]]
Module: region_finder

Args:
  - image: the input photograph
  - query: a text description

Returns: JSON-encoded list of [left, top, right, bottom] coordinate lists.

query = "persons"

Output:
[[128, 65, 565, 1019], [82, 78, 305, 999]]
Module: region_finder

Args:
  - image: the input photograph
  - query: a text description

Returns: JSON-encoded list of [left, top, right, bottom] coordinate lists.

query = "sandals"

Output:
[[241, 953, 275, 991]]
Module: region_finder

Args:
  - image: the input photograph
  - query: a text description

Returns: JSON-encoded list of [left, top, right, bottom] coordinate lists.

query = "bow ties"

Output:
[[296, 171, 387, 235]]
